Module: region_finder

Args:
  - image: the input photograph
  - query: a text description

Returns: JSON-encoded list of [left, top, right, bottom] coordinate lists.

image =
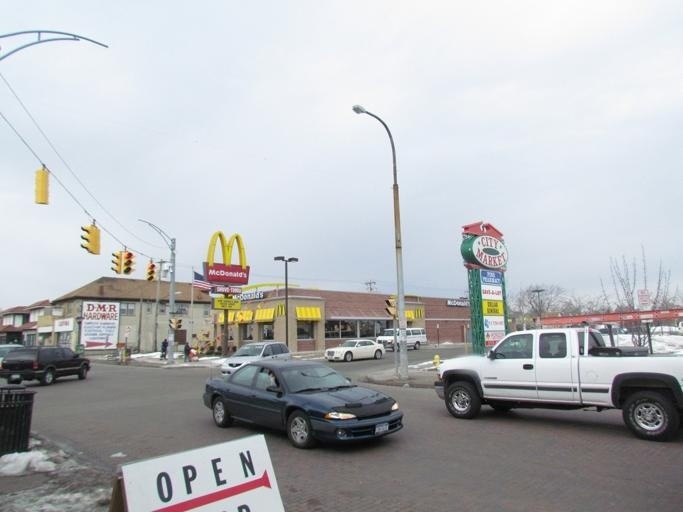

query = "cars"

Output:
[[220, 341, 293, 376], [202, 357, 404, 449], [0, 345, 25, 364], [324, 339, 386, 362]]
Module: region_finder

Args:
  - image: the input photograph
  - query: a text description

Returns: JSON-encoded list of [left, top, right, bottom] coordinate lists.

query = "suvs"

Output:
[[375, 328, 428, 351], [0, 346, 91, 384]]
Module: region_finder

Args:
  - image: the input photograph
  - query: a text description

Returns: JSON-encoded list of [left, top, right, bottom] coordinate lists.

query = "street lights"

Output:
[[138, 220, 175, 364]]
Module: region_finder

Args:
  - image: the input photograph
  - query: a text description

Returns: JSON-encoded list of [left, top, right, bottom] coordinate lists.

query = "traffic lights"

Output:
[[111, 249, 136, 276], [385, 298, 396, 316], [33, 168, 50, 206], [146, 263, 155, 282], [384, 295, 398, 320], [111, 252, 122, 274], [122, 252, 132, 274], [147, 265, 154, 282], [35, 170, 48, 204]]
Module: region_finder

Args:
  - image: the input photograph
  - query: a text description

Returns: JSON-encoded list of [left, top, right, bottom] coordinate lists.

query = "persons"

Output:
[[265, 373, 277, 391], [184, 342, 192, 362], [160, 339, 168, 360]]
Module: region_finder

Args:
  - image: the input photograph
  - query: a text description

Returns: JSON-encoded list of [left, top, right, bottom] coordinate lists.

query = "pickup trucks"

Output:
[[433, 323, 683, 442]]
